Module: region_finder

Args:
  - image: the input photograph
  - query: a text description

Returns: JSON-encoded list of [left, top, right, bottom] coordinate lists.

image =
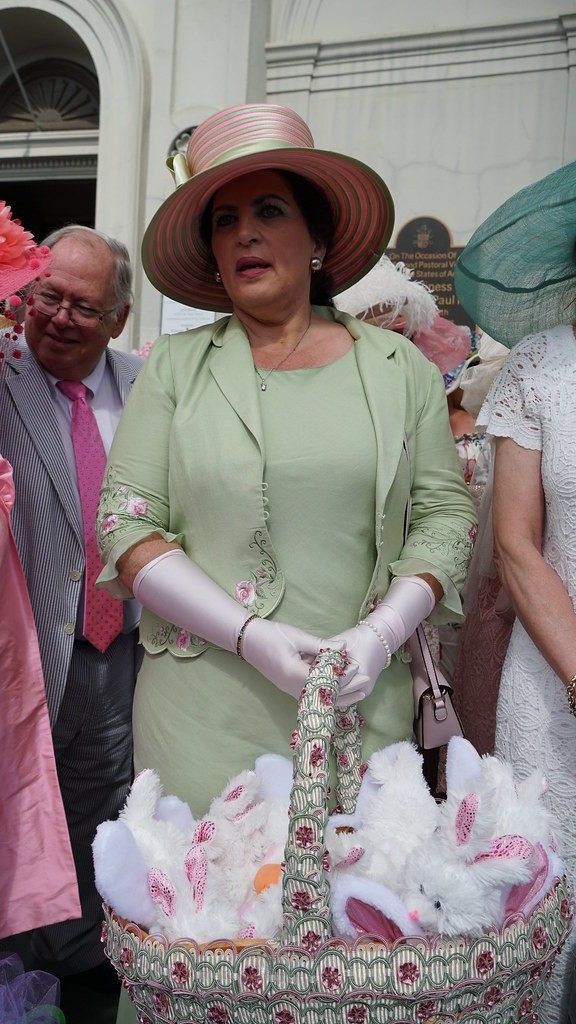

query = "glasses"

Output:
[[26, 288, 121, 328]]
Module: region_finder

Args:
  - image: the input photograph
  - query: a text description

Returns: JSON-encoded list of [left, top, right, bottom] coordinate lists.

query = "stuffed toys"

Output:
[[91, 733, 565, 946]]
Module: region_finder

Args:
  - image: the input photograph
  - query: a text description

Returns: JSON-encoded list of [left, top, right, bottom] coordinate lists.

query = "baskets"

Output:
[[101, 649, 573, 1024]]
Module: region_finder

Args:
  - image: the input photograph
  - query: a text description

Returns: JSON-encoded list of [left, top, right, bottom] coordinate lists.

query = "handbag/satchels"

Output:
[[407, 621, 465, 750]]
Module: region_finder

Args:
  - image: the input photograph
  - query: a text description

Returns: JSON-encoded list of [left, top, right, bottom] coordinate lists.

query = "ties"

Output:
[[55, 380, 122, 653]]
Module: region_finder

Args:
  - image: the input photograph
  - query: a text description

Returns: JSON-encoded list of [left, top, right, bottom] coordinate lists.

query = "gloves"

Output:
[[299, 573, 435, 699], [131, 548, 370, 706]]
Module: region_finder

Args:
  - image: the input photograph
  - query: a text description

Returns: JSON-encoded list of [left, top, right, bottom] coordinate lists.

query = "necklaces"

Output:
[[246, 309, 312, 391]]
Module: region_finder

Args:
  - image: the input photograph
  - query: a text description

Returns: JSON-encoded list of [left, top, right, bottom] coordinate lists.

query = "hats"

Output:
[[0, 198, 54, 367], [451, 161, 576, 350], [141, 103, 396, 313], [333, 254, 440, 340]]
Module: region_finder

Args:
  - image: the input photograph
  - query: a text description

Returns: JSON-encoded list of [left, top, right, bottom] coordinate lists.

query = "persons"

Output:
[[453, 161, 576, 883], [94, 103, 480, 821], [0, 226, 144, 876], [333, 259, 517, 797], [0, 201, 82, 941]]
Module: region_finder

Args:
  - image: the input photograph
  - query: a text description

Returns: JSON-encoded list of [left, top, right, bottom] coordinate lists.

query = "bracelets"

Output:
[[236, 613, 261, 660], [566, 674, 576, 717], [356, 620, 392, 669]]
